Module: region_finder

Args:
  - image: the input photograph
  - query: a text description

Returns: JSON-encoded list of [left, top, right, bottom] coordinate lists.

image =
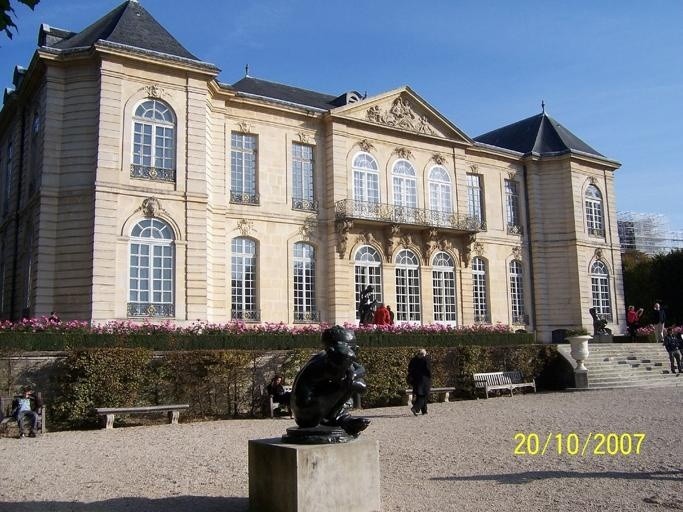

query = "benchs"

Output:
[[471, 371, 536, 399], [95, 404, 189, 429], [0, 391, 45, 438], [259, 384, 293, 419], [405, 387, 456, 411]]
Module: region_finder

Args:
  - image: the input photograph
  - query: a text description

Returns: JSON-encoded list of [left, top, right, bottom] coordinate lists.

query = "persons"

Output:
[[289, 326, 375, 434], [266, 372, 292, 417], [377, 306, 392, 326], [651, 301, 666, 343], [407, 347, 432, 416], [627, 304, 644, 341], [663, 326, 682, 373], [388, 306, 394, 323], [12, 389, 43, 439], [357, 284, 376, 321], [677, 328, 683, 372]]
